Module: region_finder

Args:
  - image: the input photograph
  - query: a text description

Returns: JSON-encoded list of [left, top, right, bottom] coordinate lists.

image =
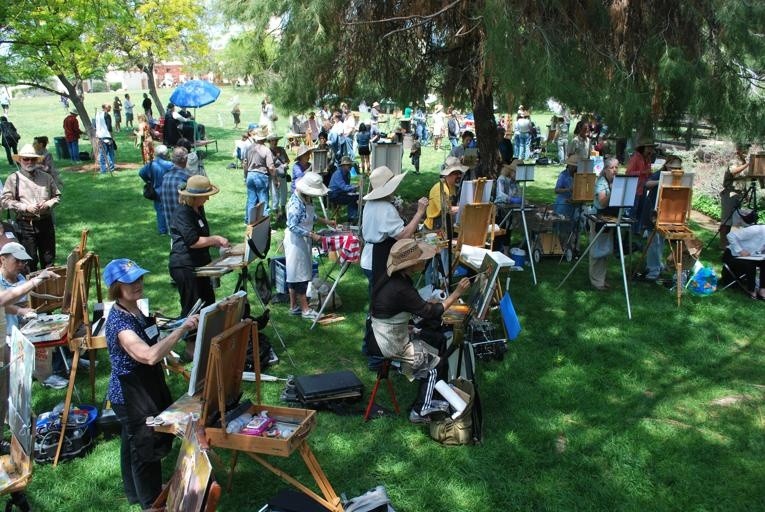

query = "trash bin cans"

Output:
[[399, 118, 412, 133], [53, 135, 68, 160]]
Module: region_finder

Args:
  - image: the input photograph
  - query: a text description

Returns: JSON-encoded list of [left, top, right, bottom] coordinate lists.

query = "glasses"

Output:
[[22, 157, 37, 161]]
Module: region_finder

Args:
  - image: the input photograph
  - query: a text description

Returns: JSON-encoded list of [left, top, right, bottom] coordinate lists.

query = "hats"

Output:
[[295, 172, 328, 197], [565, 155, 581, 166], [362, 166, 408, 200], [439, 156, 469, 175], [102, 258, 151, 288], [435, 105, 443, 113], [339, 156, 354, 165], [12, 144, 45, 162], [70, 108, 79, 115], [1, 241, 33, 261], [372, 102, 380, 107], [178, 175, 220, 197], [253, 127, 283, 140], [294, 146, 317, 161], [503, 160, 524, 172], [386, 238, 438, 277], [633, 137, 659, 149]]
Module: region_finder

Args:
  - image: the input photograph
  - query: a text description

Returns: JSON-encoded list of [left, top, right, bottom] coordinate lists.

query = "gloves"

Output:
[[17, 307, 37, 317], [32, 271, 61, 287]]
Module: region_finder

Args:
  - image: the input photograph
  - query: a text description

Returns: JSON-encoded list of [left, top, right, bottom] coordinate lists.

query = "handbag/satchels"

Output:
[[686, 266, 718, 295], [143, 182, 157, 200], [308, 282, 342, 312], [429, 378, 474, 446], [3, 219, 23, 241]]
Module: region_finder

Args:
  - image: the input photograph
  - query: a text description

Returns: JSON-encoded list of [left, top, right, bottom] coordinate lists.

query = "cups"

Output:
[[425, 232, 437, 250]]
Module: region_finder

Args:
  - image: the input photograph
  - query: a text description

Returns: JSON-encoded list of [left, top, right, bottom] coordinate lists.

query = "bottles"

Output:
[[226, 412, 252, 435]]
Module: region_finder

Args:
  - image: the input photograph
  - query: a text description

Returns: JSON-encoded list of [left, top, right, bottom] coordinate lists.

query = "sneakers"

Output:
[[410, 410, 433, 424], [179, 352, 192, 364], [290, 305, 301, 314], [302, 309, 327, 318], [41, 375, 68, 389]]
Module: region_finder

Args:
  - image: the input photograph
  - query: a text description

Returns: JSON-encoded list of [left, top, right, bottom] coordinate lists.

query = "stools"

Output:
[[328, 202, 345, 222], [190, 137, 223, 156], [363, 349, 406, 419]]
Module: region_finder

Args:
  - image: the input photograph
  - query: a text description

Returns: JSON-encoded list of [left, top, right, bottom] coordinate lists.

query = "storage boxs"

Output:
[[270, 253, 321, 305]]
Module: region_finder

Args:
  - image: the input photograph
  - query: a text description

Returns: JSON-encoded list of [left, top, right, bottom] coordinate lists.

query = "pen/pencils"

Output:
[[426, 196, 434, 202], [449, 270, 486, 287], [493, 275, 512, 339], [36, 273, 69, 281], [22, 299, 49, 319]]
